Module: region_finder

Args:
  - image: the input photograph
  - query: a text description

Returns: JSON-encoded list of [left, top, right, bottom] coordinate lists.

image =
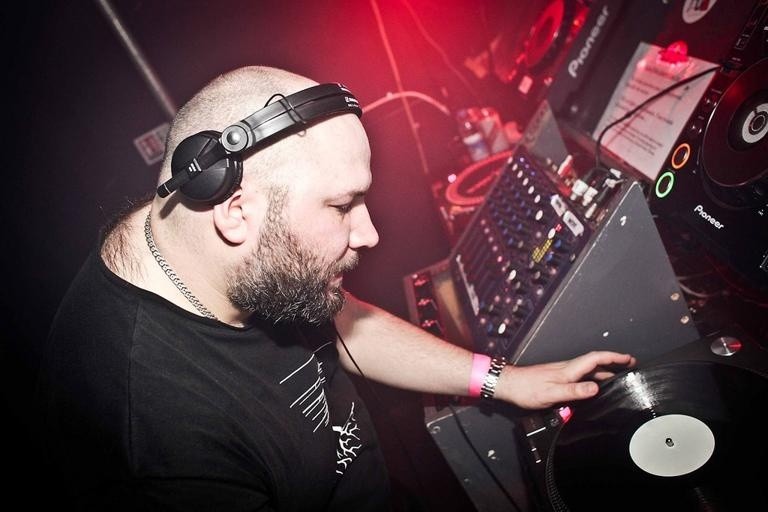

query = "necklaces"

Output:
[[144, 209, 217, 321]]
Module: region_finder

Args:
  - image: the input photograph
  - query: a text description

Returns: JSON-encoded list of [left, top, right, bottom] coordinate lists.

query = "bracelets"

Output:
[[479, 353, 509, 402], [469, 352, 491, 399]]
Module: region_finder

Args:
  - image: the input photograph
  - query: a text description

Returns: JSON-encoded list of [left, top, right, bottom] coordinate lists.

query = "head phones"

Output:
[[157, 82, 362, 207]]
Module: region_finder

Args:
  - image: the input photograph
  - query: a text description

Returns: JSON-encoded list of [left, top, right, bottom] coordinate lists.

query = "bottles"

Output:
[[456, 106, 511, 165]]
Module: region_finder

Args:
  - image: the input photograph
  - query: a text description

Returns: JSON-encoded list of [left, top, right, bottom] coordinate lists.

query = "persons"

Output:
[[19, 65, 639, 507]]
